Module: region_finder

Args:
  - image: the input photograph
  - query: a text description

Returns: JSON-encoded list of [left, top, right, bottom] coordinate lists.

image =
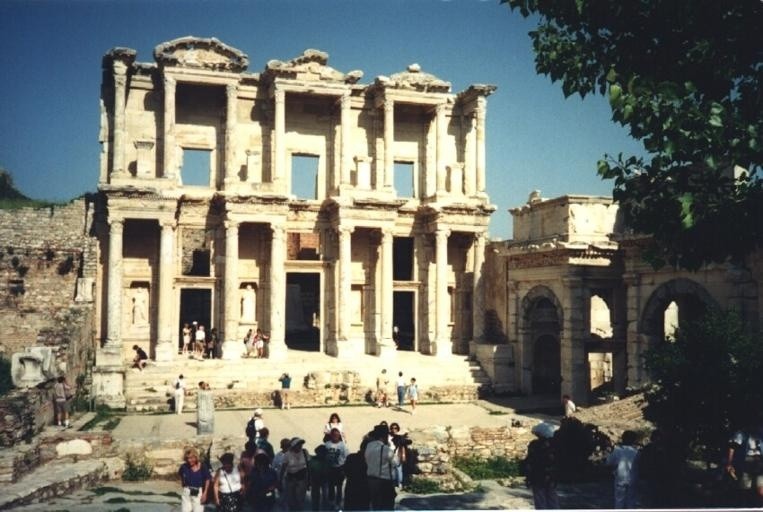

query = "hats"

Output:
[[252, 448, 267, 460], [279, 437, 306, 454]]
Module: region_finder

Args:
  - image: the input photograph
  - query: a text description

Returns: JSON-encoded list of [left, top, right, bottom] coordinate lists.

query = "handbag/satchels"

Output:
[[366, 477, 396, 500]]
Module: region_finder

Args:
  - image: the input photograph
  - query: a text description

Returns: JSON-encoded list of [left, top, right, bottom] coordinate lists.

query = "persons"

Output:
[[526, 424, 560, 509], [562, 394, 575, 418], [376, 368, 390, 408], [53, 377, 71, 426], [172, 374, 185, 414], [132, 345, 148, 370], [181, 321, 268, 361], [177, 409, 407, 511], [604, 412, 762, 507], [395, 371, 407, 409], [198, 382, 211, 391], [278, 373, 292, 410], [406, 378, 418, 417]]
